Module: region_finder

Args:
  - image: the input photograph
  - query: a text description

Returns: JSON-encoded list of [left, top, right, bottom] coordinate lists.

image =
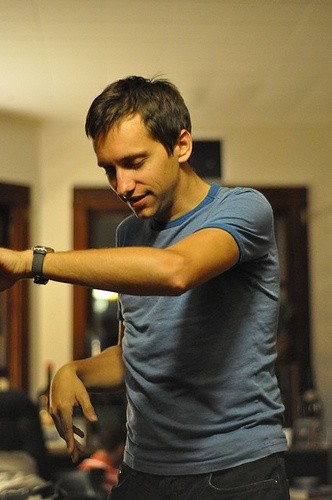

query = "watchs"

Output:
[[32, 245, 54, 285]]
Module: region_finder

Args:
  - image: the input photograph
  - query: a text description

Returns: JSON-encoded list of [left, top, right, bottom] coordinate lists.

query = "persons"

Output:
[[0, 367, 126, 500], [0, 75, 291, 500]]
